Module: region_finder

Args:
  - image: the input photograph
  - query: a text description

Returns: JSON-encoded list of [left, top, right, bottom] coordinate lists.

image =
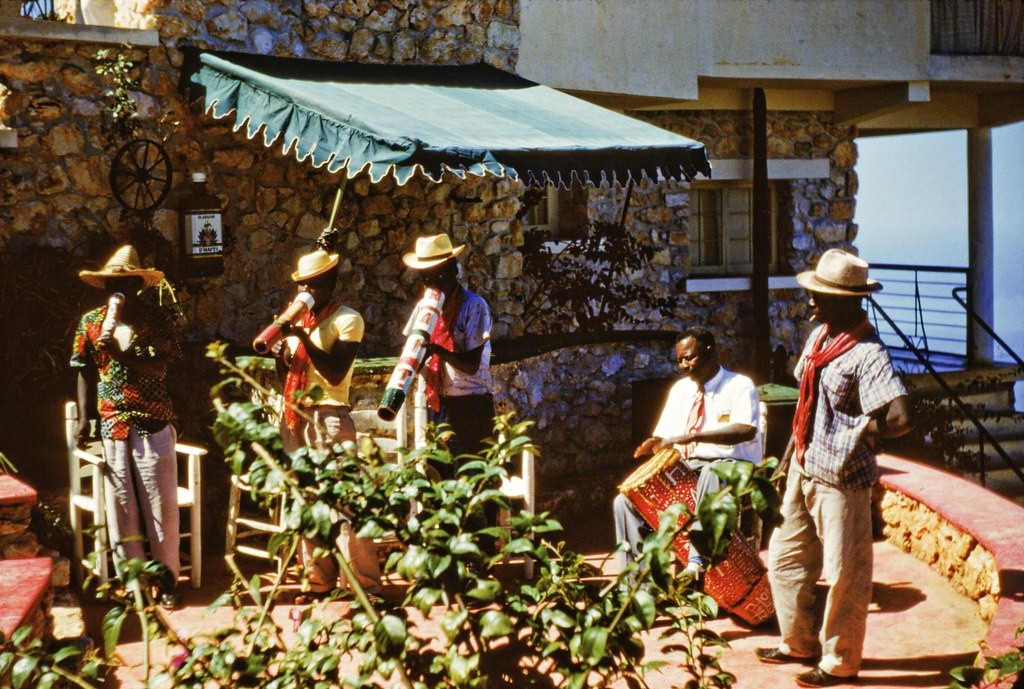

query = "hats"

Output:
[[291, 251, 340, 282], [402, 234, 465, 271], [796, 248, 883, 297], [79, 244, 165, 293]]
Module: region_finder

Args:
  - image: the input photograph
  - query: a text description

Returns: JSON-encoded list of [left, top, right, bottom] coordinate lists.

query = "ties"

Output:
[[684, 385, 705, 458]]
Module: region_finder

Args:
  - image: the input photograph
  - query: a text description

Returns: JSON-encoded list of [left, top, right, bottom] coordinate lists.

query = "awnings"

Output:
[[179, 47, 712, 188]]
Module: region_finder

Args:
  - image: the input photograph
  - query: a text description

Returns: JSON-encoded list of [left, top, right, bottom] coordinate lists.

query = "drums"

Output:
[[618, 447, 776, 626]]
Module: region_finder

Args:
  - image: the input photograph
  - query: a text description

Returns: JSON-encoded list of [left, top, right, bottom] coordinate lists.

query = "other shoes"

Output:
[[294, 587, 337, 605], [755, 647, 821, 663], [160, 588, 178, 609], [354, 592, 386, 606], [794, 667, 857, 687]]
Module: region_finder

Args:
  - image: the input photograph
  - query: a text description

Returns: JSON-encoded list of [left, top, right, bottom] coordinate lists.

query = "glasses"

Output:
[[425, 274, 445, 283]]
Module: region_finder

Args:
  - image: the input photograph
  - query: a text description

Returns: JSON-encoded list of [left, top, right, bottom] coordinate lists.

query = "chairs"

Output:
[[222, 473, 292, 584], [498, 441, 536, 580], [63, 400, 208, 600], [349, 392, 409, 474]]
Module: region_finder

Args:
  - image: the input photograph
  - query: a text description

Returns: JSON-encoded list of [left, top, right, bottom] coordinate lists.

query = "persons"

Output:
[[754, 249, 916, 688], [272, 250, 384, 609], [402, 234, 497, 577], [613, 327, 763, 597], [69, 246, 183, 610]]
